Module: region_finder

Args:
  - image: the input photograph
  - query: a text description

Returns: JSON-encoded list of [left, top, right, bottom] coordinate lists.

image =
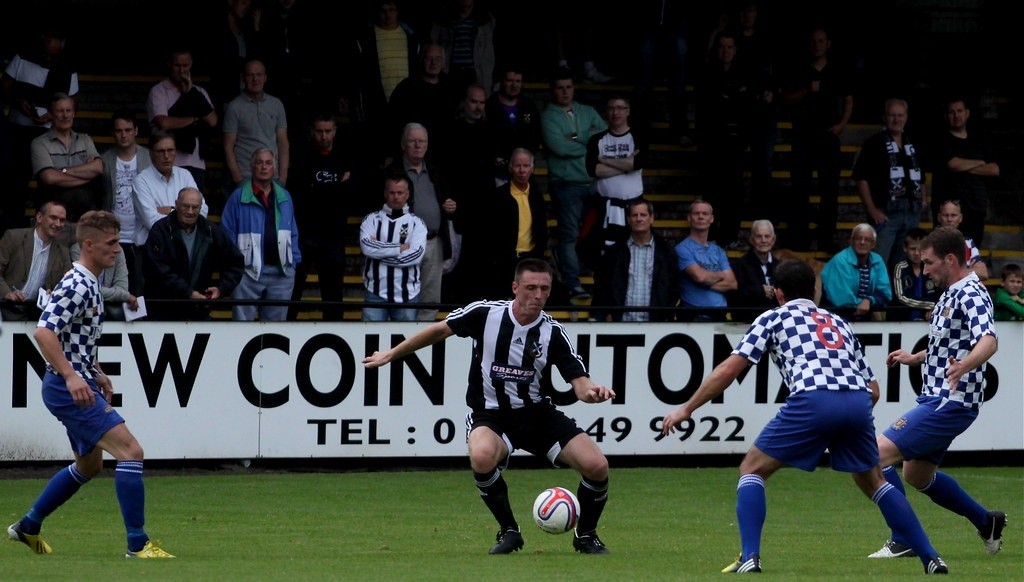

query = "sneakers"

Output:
[[8, 521, 52, 554], [573, 527, 609, 554], [977, 510, 1007, 555], [721, 552, 761, 573], [928, 557, 948, 574], [489, 525, 524, 554], [868, 537, 918, 558], [125, 541, 176, 558]]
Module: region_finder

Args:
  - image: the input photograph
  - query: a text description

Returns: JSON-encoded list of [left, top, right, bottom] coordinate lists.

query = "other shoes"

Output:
[[569, 286, 590, 298], [729, 240, 750, 251]]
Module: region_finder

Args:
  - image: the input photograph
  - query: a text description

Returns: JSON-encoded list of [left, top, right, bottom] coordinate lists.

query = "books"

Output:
[[122, 296, 147, 322]]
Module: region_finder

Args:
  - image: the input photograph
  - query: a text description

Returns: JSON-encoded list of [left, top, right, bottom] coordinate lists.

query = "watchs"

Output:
[[62, 168, 68, 174]]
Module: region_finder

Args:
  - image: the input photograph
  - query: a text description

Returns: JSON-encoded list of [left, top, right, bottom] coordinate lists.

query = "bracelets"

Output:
[[571, 132, 578, 143], [193, 117, 197, 124]]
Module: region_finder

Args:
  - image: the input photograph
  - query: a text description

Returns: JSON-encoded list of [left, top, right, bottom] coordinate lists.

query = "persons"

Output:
[[852, 99, 929, 284], [362, 257, 617, 556], [28, 91, 106, 230], [488, 147, 550, 311], [130, 133, 209, 321], [661, 257, 949, 575], [817, 223, 892, 322], [867, 225, 1007, 559], [665, 199, 738, 323], [219, 147, 302, 322], [359, 172, 429, 322], [222, 59, 290, 206], [141, 186, 245, 322], [993, 263, 1024, 322], [592, 197, 680, 323], [7, 210, 178, 560], [0, 0, 1024, 322]]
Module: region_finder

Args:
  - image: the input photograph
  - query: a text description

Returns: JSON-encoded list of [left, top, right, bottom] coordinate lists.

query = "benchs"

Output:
[[23, 74, 1024, 318]]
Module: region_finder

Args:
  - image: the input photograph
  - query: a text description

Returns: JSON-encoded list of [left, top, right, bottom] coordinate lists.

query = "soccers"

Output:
[[532, 486, 582, 535]]
